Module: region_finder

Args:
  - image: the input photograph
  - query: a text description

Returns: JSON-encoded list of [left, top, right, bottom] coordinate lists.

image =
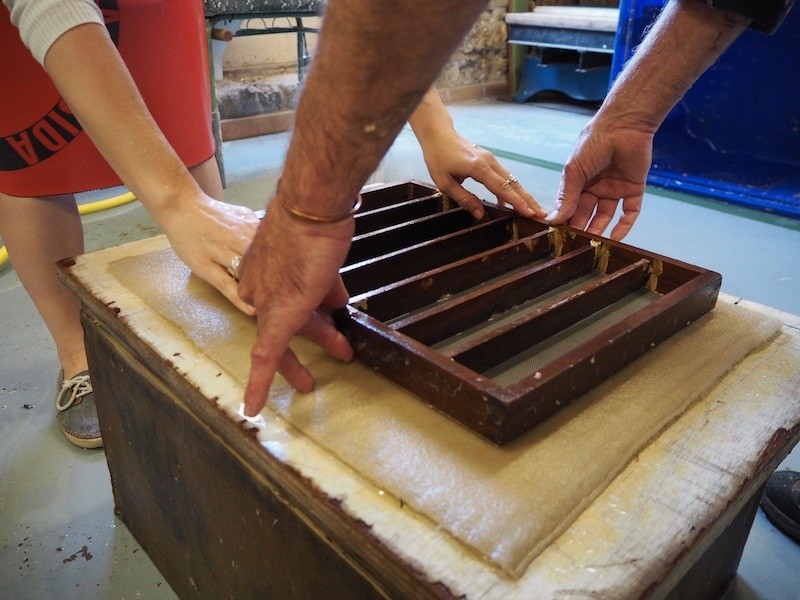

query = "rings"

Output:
[[227, 254, 242, 276], [505, 174, 517, 184]]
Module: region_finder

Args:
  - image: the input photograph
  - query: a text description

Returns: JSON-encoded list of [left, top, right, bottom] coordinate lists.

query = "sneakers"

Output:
[[56, 366, 104, 448]]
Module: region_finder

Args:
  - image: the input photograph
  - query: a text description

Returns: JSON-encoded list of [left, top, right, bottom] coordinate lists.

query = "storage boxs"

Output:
[[607, 0, 800, 221]]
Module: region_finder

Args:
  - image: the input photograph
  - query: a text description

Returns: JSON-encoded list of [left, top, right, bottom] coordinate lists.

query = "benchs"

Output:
[[505, 6, 620, 109]]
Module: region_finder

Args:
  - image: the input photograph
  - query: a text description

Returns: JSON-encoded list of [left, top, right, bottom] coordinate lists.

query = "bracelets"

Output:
[[276, 174, 363, 222]]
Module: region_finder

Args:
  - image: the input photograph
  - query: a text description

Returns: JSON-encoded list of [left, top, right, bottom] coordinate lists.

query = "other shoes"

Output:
[[760, 469, 800, 543]]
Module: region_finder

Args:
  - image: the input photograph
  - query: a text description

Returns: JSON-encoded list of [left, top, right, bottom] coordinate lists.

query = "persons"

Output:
[[236, 0, 800, 540], [0, 0, 262, 448]]
